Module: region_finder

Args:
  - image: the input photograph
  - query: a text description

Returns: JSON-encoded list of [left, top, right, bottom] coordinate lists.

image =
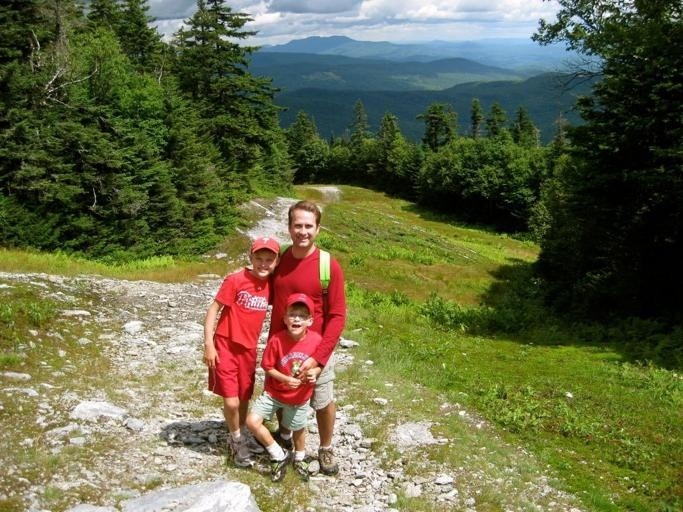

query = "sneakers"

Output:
[[318, 445, 338, 475], [268, 429, 309, 482], [227, 426, 264, 467]]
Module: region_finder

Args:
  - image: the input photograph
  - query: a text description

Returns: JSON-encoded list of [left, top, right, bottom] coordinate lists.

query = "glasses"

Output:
[[287, 311, 308, 319]]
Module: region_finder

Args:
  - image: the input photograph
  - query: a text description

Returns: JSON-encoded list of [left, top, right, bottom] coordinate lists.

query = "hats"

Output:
[[287, 293, 314, 317], [251, 237, 280, 253]]
